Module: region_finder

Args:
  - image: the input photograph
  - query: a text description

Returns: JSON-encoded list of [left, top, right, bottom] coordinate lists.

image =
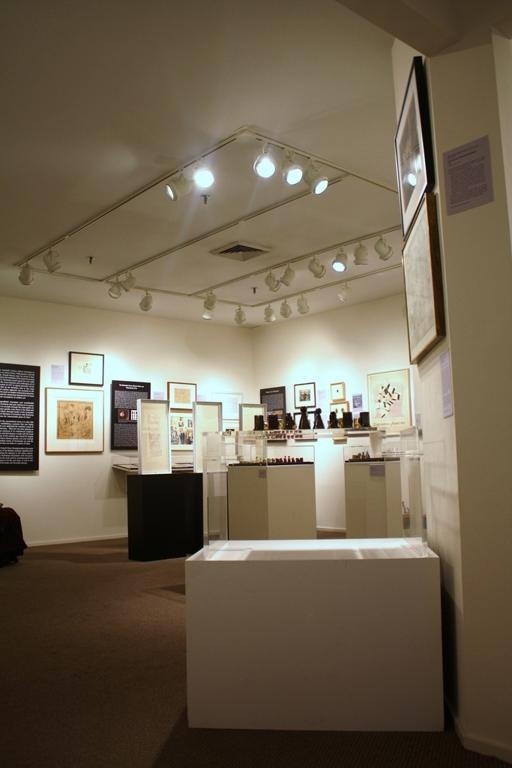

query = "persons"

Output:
[[171, 416, 193, 444]]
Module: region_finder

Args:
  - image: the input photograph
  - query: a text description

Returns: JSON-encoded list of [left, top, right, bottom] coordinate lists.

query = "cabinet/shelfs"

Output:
[[182, 538, 445, 734]]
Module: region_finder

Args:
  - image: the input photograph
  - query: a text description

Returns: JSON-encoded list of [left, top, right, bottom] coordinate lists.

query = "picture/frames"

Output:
[[69, 351, 105, 386], [167, 381, 197, 408], [170, 413, 193, 451], [293, 383, 316, 407], [135, 398, 172, 475], [400, 190, 447, 365], [44, 386, 105, 453], [194, 401, 223, 471], [239, 403, 267, 431], [293, 412, 315, 429], [394, 54, 436, 242], [329, 382, 345, 401], [367, 368, 412, 427], [330, 401, 349, 421]]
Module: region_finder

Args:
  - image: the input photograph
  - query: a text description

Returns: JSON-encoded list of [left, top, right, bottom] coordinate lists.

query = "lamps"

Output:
[[13, 122, 402, 331]]
